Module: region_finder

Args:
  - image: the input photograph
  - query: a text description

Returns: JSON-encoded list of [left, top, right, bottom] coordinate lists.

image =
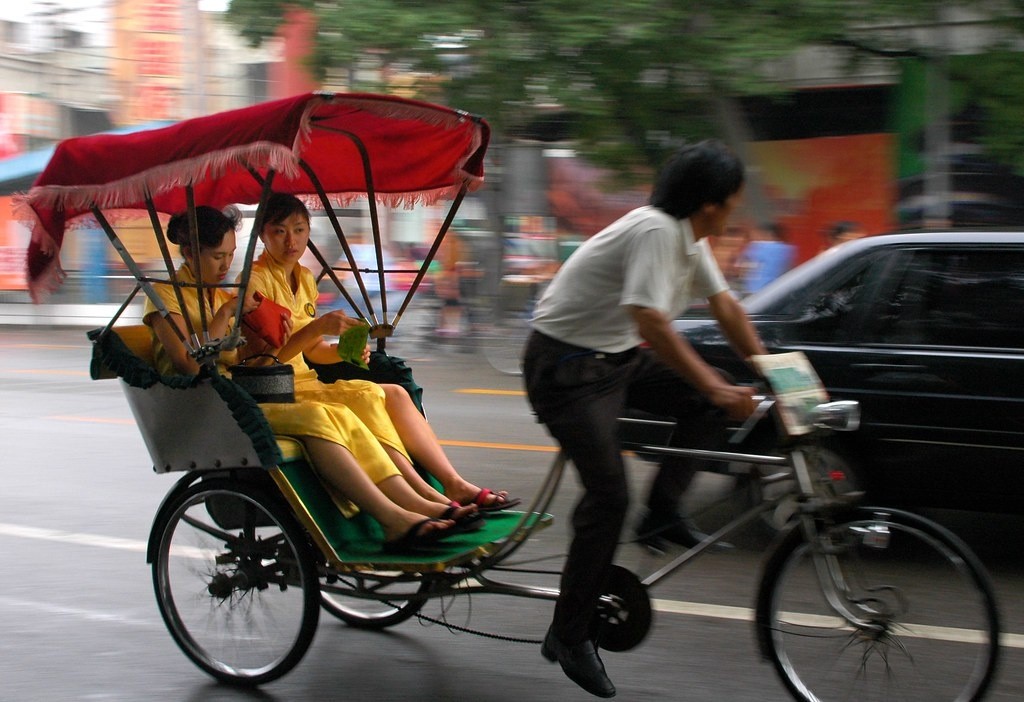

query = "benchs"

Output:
[[88, 323, 555, 574]]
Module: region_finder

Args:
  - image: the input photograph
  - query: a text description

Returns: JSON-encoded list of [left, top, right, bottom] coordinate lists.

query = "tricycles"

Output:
[[24, 90, 1003, 702]]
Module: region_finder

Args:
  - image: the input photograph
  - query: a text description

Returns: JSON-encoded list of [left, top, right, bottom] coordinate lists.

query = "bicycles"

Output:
[[399, 293, 534, 380]]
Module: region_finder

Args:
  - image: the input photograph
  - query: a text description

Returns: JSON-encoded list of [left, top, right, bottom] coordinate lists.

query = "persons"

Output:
[[735, 208, 796, 296], [823, 220, 865, 251], [522, 138, 772, 697], [144, 192, 522, 545], [322, 224, 397, 330]]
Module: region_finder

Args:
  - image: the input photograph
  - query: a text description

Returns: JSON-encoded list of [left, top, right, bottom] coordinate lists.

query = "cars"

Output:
[[604, 229, 1022, 549]]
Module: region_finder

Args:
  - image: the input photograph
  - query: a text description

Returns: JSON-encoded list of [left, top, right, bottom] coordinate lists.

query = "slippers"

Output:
[[475, 486, 522, 511], [441, 506, 481, 535], [449, 501, 462, 509], [384, 516, 460, 551]]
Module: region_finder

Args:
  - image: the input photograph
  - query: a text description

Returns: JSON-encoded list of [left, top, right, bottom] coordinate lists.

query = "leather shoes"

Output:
[[635, 512, 734, 552], [541, 623, 615, 698]]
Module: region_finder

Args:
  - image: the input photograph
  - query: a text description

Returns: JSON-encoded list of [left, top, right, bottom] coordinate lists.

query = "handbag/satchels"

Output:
[[227, 352, 295, 404]]
